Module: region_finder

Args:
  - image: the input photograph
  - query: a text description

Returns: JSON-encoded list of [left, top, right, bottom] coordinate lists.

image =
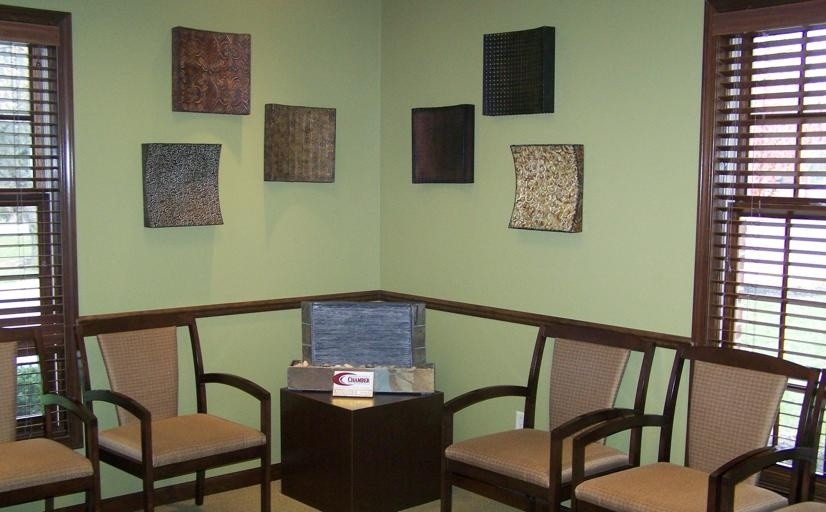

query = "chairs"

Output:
[[2, 326, 102, 510], [434, 322, 659, 510], [572, 343, 823, 510], [74, 309, 274, 509], [720, 368, 824, 510]]
